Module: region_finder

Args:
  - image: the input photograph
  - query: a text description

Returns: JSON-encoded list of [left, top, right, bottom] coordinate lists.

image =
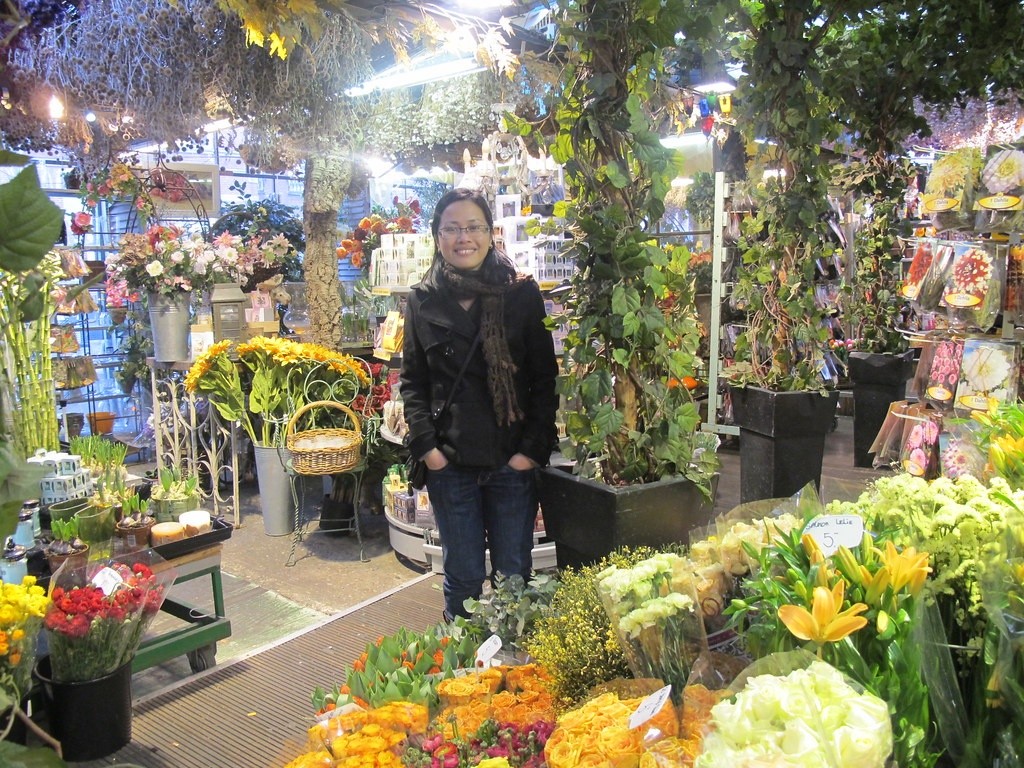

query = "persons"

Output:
[[399, 189, 562, 625]]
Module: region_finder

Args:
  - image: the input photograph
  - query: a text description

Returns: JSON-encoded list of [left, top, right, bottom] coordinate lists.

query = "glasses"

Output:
[[439, 224, 491, 238]]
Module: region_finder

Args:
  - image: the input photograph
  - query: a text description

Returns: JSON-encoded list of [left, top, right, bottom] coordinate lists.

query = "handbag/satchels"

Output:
[[404, 401, 448, 496]]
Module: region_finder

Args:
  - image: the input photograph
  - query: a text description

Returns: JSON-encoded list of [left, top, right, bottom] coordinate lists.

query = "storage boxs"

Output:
[[248, 322, 280, 341], [27, 449, 92, 505], [394, 493, 416, 524], [381, 476, 407, 515], [84, 261, 107, 284], [369, 194, 574, 286]]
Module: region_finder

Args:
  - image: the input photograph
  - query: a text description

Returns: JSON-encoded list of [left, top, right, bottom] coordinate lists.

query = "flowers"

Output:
[[0, 1, 565, 173], [352, 361, 405, 472], [186, 335, 371, 448], [336, 195, 421, 271], [660, 240, 713, 293], [70, 165, 305, 309], [284, 397, 1024, 768], [830, 338, 864, 366], [0, 560, 164, 715]]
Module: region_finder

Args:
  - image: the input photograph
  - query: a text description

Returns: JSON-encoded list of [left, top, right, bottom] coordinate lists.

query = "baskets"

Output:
[[286, 401, 362, 476]]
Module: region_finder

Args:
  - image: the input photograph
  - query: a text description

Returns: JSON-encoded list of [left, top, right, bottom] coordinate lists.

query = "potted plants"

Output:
[[496, 1, 1024, 581], [44, 518, 90, 589], [92, 462, 200, 554]]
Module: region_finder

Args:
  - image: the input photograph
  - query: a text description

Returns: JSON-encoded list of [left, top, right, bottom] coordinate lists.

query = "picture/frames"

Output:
[[142, 164, 221, 217]]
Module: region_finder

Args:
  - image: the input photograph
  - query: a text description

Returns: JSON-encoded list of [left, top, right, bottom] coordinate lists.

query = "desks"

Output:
[[24, 542, 231, 706], [144, 342, 372, 530]]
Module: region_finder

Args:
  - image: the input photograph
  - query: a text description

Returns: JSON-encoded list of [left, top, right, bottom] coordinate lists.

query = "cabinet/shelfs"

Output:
[[373, 286, 570, 571], [47, 284, 146, 462], [565, 219, 723, 437]]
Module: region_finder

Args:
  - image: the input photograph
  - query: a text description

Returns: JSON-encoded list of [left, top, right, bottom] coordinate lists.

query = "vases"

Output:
[[147, 290, 191, 363], [74, 506, 117, 544], [32, 655, 136, 763], [64, 175, 81, 188], [62, 414, 84, 438], [113, 370, 137, 393], [107, 306, 128, 325], [48, 497, 88, 522], [252, 441, 301, 537], [86, 412, 115, 436]]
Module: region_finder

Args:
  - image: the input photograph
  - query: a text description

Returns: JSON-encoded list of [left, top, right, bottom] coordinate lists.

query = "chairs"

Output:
[[262, 357, 384, 567]]
